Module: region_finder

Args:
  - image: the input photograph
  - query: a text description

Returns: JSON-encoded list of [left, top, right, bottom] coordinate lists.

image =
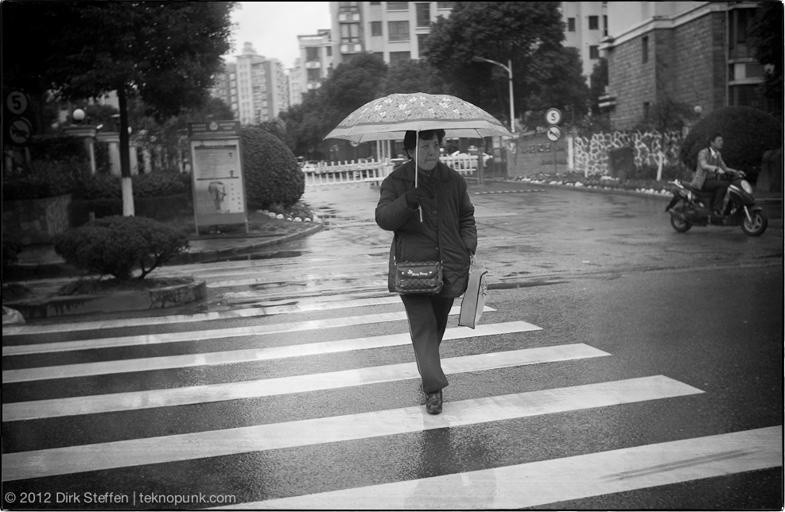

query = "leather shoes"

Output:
[[426, 392, 442, 414]]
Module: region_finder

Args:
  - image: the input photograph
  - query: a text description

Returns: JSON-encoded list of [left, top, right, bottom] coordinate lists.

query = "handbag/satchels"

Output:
[[458, 249, 488, 329], [396, 262, 442, 293]]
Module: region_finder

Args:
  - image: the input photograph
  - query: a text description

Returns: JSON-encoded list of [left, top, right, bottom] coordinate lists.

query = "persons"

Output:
[[692, 133, 746, 216], [375, 130, 478, 414]]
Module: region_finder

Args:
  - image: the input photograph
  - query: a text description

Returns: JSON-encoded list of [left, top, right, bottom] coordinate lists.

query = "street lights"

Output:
[[472, 55, 515, 133]]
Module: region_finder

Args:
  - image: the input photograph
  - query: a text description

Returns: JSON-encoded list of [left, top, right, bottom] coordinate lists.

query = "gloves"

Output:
[[406, 189, 420, 208]]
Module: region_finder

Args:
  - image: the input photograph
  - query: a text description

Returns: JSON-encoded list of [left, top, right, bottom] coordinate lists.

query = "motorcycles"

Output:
[[666, 173, 768, 237]]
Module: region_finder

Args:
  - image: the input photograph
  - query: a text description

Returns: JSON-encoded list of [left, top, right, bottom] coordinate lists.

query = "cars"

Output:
[[447, 148, 493, 169], [303, 160, 317, 168]]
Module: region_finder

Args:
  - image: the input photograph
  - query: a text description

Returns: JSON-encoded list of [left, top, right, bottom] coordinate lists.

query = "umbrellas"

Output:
[[321, 93, 514, 187]]
